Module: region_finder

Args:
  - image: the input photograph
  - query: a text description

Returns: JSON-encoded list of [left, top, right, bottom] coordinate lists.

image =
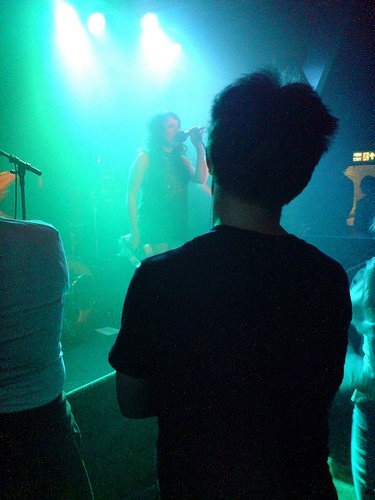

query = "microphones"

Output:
[[176, 125, 207, 142]]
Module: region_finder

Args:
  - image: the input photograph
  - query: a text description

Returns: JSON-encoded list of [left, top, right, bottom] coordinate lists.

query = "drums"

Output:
[[64, 271, 99, 340]]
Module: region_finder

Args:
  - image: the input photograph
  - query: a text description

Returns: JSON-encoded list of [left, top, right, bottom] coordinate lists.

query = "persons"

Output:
[[108, 67, 352, 500], [128, 112, 208, 264], [1, 212, 98, 500], [352, 174, 375, 234], [342, 218, 375, 500]]
